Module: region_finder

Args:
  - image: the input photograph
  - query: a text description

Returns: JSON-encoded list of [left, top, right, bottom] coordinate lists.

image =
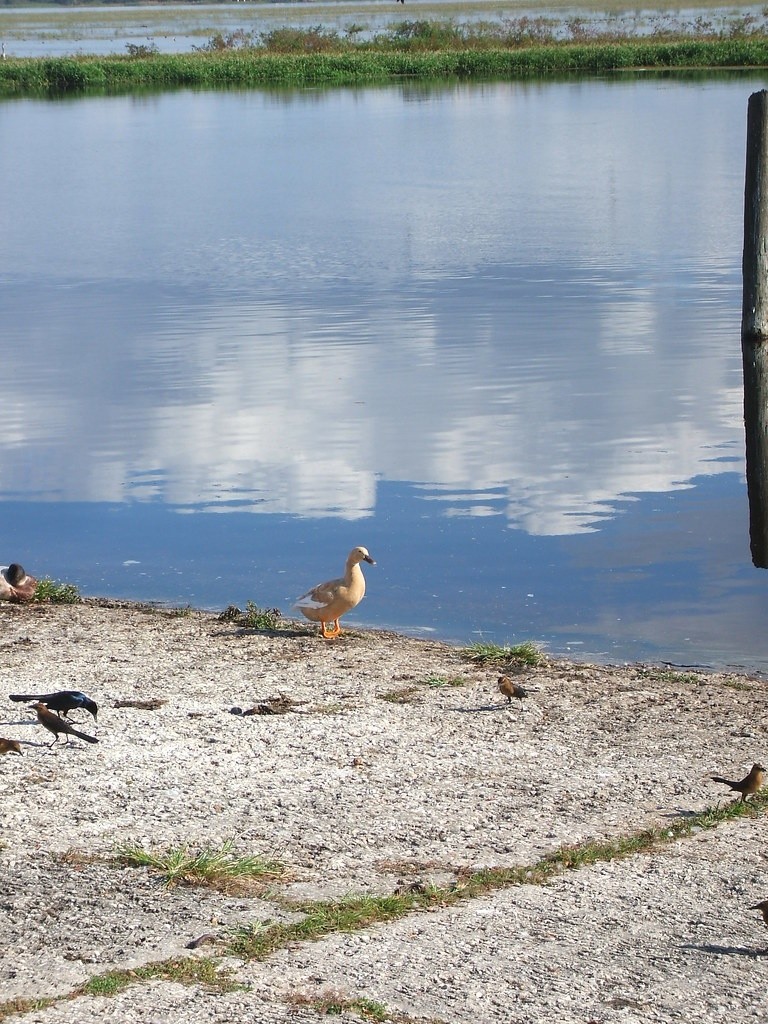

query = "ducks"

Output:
[[289, 546, 377, 638]]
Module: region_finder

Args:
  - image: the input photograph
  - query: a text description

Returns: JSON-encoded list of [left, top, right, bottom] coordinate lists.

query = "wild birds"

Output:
[[8, 690, 99, 723], [497, 676, 540, 704], [27, 703, 100, 747], [711, 764, 766, 807], [0, 737, 24, 758], [0, 563, 38, 601]]
